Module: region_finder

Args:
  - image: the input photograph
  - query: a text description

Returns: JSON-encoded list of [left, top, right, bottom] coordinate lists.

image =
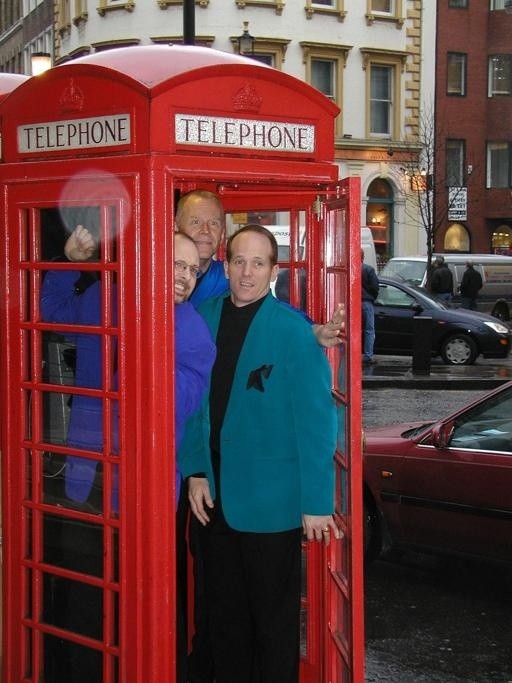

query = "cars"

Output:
[[374, 278, 512, 365], [363, 378, 511, 565]]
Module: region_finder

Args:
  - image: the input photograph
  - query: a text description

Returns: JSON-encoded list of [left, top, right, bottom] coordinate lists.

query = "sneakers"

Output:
[[365, 359, 378, 365]]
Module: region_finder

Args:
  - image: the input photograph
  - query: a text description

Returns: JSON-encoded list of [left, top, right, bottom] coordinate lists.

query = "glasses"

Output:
[[174, 260, 203, 279]]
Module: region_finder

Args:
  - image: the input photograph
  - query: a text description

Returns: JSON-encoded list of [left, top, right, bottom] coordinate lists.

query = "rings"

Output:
[[323, 528, 330, 532]]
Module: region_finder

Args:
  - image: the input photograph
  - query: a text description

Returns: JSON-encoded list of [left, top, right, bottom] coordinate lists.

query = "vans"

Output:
[[263, 225, 376, 296], [376, 253, 512, 322]]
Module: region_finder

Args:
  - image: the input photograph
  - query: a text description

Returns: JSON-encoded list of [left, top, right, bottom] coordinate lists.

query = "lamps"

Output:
[[237, 20, 255, 56]]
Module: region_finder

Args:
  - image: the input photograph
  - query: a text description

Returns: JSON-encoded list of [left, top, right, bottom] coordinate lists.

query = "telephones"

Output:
[[49, 342, 76, 445]]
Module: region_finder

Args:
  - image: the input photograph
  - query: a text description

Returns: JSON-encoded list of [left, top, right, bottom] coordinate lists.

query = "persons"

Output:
[[361, 247, 379, 365], [276, 267, 307, 311], [37, 226, 217, 681], [181, 224, 344, 682], [430, 256, 483, 311], [175, 191, 344, 349]]
